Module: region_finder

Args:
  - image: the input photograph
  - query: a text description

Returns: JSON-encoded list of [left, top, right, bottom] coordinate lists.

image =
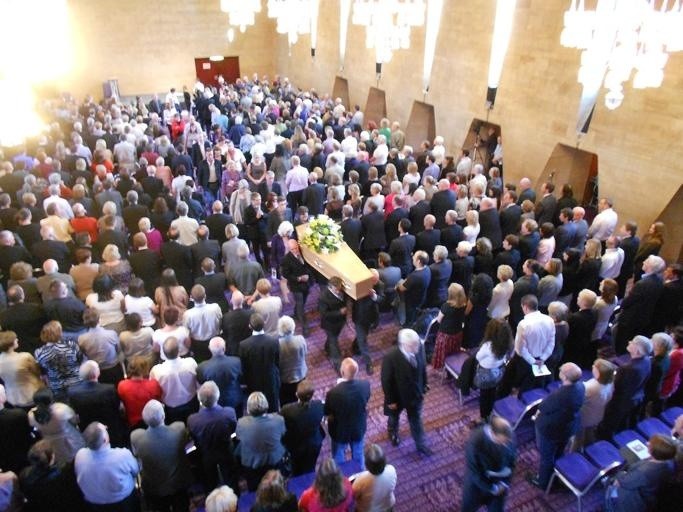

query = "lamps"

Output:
[[220, 1, 683, 135]]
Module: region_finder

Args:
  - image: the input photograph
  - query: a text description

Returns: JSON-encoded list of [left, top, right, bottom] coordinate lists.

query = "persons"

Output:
[[0, 92, 309, 423], [0, 357, 398, 511], [362, 120, 538, 327], [461, 419, 515, 511], [424, 184, 683, 417], [381, 328, 434, 455], [610, 415, 682, 511], [166, 74, 378, 374], [523, 327, 683, 488]]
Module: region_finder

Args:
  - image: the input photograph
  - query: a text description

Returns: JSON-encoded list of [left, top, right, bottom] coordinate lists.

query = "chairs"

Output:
[[409, 296, 683, 512], [132, 428, 366, 512]]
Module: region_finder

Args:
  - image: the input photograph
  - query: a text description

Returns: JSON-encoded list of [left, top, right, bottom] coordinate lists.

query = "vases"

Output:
[[320, 246, 331, 255]]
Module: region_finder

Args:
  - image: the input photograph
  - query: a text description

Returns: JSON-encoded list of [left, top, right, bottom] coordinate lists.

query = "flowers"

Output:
[[298, 214, 343, 250]]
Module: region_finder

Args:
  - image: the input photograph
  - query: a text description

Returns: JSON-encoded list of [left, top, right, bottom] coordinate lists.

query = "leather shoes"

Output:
[[389, 433, 399, 446], [367, 364, 373, 375]]
